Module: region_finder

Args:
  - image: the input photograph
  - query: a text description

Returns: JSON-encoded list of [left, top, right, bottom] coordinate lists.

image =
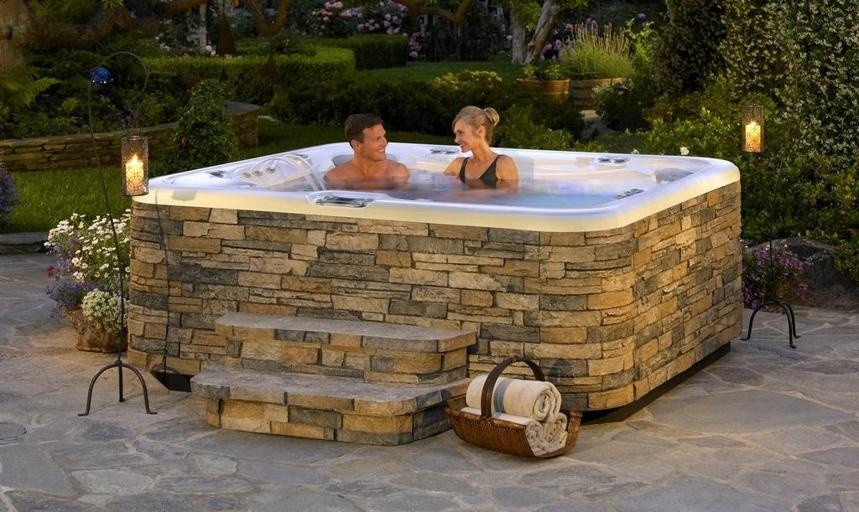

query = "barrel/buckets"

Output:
[[521, 78, 569, 104]]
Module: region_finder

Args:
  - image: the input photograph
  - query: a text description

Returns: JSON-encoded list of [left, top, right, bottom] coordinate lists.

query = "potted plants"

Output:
[[516, 55, 569, 104]]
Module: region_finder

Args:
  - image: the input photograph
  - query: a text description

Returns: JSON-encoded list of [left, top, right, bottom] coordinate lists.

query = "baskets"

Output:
[[442, 354, 582, 460]]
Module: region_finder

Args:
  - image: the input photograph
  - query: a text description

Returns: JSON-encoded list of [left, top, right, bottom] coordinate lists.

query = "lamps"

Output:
[[740, 33, 801, 348], [78, 49, 155, 416]]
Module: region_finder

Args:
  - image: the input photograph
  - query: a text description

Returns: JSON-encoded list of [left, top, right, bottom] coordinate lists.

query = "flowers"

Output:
[[43, 208, 130, 336], [555, 17, 639, 78]]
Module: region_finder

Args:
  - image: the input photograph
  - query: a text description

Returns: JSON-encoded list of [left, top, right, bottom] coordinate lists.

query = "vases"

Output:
[[571, 79, 624, 110], [68, 311, 128, 353]]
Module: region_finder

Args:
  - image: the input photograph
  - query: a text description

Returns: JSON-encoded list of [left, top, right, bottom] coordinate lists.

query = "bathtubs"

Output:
[[129, 141, 740, 233]]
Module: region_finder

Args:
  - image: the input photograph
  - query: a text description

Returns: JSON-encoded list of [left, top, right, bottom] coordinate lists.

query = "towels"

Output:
[[461, 375, 567, 456]]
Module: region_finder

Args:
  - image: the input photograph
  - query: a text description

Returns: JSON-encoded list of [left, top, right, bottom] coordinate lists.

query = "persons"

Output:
[[443, 106, 520, 198], [324, 112, 410, 193]]
